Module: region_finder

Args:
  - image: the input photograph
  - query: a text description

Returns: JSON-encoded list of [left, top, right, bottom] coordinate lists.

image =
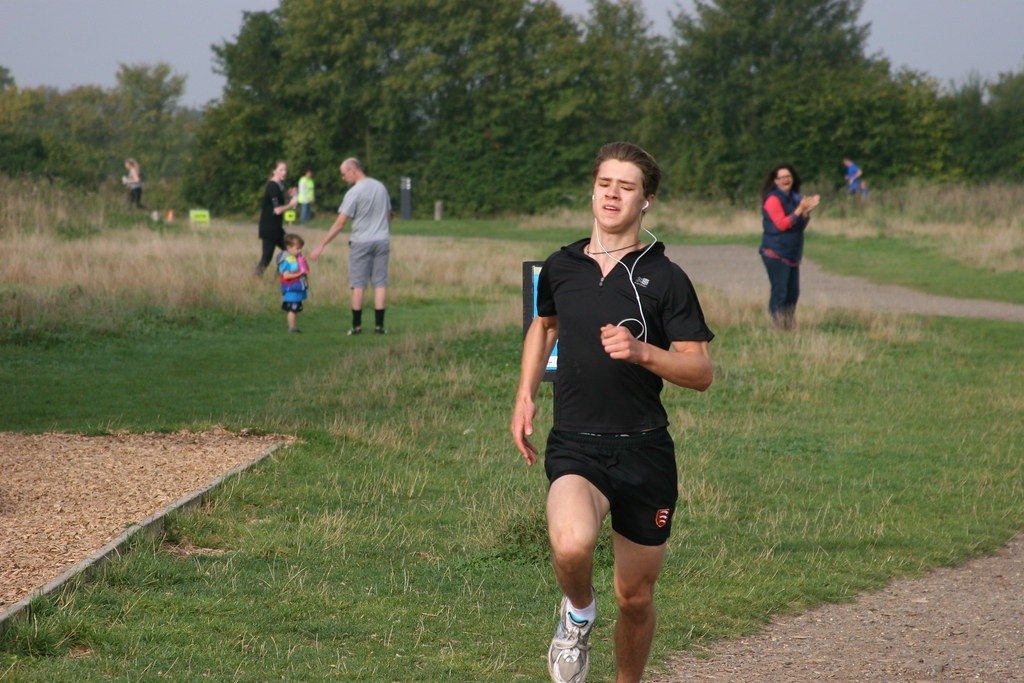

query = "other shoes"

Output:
[[289, 328, 302, 333], [374, 328, 387, 334], [347, 328, 362, 335]]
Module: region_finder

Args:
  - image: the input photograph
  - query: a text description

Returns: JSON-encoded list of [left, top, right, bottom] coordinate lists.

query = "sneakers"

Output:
[[546, 585, 597, 682]]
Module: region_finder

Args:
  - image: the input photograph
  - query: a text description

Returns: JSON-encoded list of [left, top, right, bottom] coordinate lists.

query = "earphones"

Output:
[[641, 200, 650, 210], [592, 194, 595, 201]]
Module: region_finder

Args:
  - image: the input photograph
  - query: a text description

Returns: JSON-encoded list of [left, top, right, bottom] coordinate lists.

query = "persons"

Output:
[[296, 169, 315, 228], [757, 160, 820, 333], [251, 156, 298, 283], [856, 179, 873, 209], [277, 234, 310, 334], [285, 183, 315, 225], [841, 151, 863, 226], [509, 141, 715, 683], [309, 156, 393, 336], [123, 158, 147, 212]]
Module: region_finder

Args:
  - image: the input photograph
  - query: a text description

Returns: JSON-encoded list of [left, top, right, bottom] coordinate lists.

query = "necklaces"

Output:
[[586, 240, 641, 255]]
